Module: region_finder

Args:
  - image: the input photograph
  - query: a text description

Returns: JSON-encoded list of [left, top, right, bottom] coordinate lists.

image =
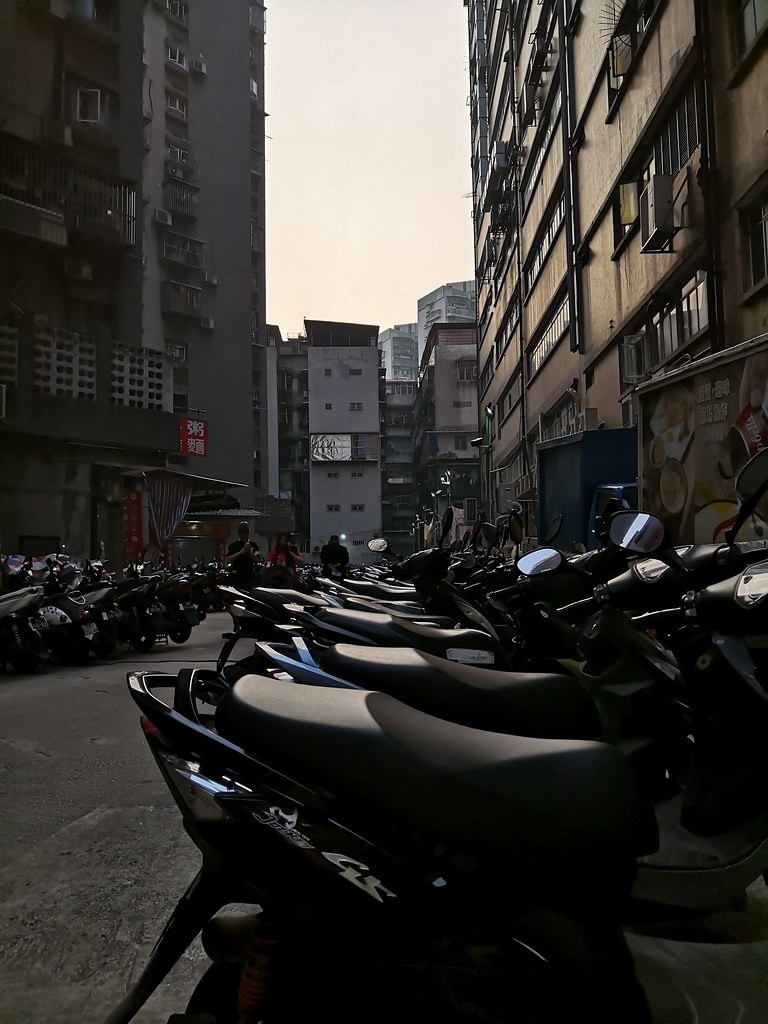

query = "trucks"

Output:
[[535, 427, 639, 554]]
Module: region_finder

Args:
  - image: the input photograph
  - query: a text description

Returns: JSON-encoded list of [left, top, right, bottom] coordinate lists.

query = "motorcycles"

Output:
[[0, 545, 356, 675], [126, 447, 768, 1024]]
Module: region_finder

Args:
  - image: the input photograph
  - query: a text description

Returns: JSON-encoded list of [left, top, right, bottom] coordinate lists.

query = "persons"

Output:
[[266, 532, 302, 585], [320, 535, 349, 584], [225, 521, 259, 590]]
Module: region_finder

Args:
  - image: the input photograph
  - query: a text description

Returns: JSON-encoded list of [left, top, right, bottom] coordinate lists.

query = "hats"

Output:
[[330, 535, 339, 541]]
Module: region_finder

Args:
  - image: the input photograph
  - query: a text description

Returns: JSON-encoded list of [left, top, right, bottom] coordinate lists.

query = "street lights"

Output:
[[409, 468, 453, 552], [485, 405, 496, 525]]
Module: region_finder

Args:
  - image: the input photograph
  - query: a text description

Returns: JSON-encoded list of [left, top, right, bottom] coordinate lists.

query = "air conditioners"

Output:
[[531, 37, 547, 66], [521, 82, 535, 126], [491, 141, 506, 172], [190, 59, 207, 75], [312, 544, 322, 552], [477, 57, 487, 81], [462, 498, 477, 523], [495, 484, 516, 514], [153, 207, 173, 227], [200, 317, 215, 329], [62, 258, 92, 282], [639, 174, 674, 250]]
